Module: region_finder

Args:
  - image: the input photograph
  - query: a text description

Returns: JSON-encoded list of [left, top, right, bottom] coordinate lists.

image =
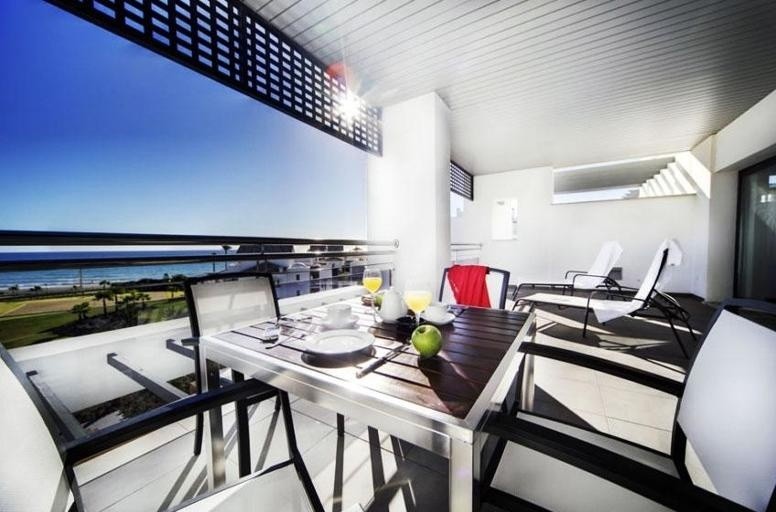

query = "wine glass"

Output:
[[404, 279, 434, 344], [362, 268, 382, 315]]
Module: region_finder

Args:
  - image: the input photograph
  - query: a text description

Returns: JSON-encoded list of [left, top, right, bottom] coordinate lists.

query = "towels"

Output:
[[592, 238, 682, 324], [575, 241, 624, 288]]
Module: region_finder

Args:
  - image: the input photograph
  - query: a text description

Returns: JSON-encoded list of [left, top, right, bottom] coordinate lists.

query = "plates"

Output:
[[423, 312, 456, 326], [321, 314, 359, 328], [303, 328, 376, 355]]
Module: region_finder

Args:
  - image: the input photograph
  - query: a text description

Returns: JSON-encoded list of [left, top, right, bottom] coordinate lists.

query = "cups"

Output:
[[427, 300, 451, 320], [328, 304, 352, 322]]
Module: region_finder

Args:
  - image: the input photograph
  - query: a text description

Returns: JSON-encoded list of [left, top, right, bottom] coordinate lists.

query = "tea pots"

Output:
[[371, 286, 408, 324]]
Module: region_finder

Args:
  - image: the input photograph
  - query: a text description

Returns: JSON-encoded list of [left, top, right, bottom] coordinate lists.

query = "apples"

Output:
[[411, 324, 443, 359], [374, 295, 383, 306]]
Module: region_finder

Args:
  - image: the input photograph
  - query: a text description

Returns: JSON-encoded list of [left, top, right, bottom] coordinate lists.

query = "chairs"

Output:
[[0, 342, 324, 512], [438, 267, 510, 415], [512, 238, 698, 359], [512, 241, 627, 301], [473, 305, 776, 512], [184, 272, 344, 480]]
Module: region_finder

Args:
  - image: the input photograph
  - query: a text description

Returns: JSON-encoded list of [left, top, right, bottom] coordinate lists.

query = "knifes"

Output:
[[356, 344, 409, 378]]
